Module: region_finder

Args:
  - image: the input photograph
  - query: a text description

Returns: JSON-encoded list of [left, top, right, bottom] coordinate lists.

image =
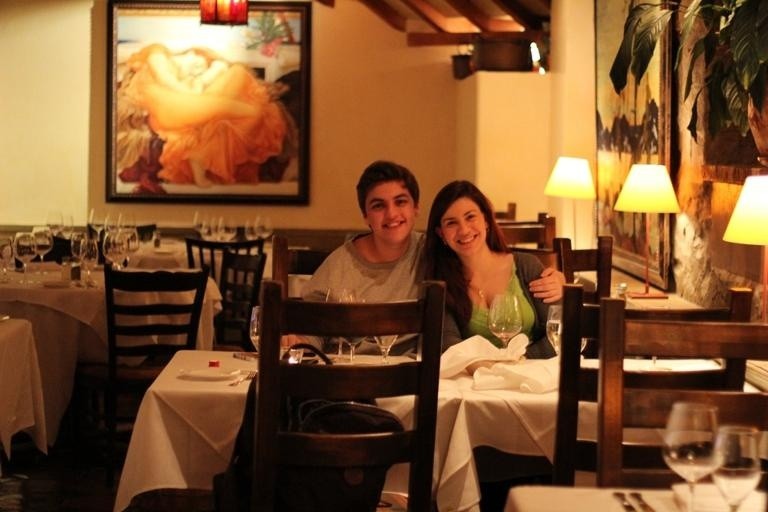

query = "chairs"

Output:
[[1, 203, 767, 510]]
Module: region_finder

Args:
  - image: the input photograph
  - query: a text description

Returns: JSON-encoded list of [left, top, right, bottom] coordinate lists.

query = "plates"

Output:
[[178, 367, 240, 380]]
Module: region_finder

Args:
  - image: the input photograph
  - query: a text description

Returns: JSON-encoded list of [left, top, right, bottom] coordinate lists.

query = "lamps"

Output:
[[612, 163, 679, 298], [199, 0, 249, 28], [545, 156, 596, 249], [722, 175, 768, 324]]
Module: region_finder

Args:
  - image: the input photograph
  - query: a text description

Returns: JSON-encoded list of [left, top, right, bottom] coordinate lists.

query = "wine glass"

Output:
[[711, 424, 761, 511], [250, 287, 401, 369], [45, 207, 140, 287], [488, 295, 524, 348], [0, 237, 13, 284], [547, 305, 587, 356], [660, 403, 720, 511], [13, 232, 37, 284], [193, 210, 273, 242], [31, 227, 54, 263]]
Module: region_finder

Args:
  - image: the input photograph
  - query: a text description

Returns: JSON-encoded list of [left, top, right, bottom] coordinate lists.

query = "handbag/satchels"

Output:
[[213, 340, 403, 511]]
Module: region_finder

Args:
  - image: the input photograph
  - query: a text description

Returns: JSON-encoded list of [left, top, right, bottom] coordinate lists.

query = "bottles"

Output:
[[153, 231, 160, 248], [614, 283, 628, 307]]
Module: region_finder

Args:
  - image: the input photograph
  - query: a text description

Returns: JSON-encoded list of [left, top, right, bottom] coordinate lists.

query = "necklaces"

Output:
[[466, 261, 500, 299]]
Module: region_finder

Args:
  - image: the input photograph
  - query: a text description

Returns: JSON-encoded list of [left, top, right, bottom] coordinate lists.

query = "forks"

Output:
[[231, 371, 255, 388]]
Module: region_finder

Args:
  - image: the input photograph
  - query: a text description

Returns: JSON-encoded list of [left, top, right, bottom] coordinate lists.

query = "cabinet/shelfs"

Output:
[[453, 72, 548, 253]]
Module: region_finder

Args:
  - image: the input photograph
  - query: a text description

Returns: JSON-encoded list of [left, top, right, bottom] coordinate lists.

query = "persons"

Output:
[[281, 159, 566, 354], [415, 179, 564, 358], [130, 47, 263, 131]]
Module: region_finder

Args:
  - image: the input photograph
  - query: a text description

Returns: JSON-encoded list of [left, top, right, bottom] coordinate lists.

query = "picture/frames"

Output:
[[594, 1, 680, 292], [105, 0, 312, 207]]
